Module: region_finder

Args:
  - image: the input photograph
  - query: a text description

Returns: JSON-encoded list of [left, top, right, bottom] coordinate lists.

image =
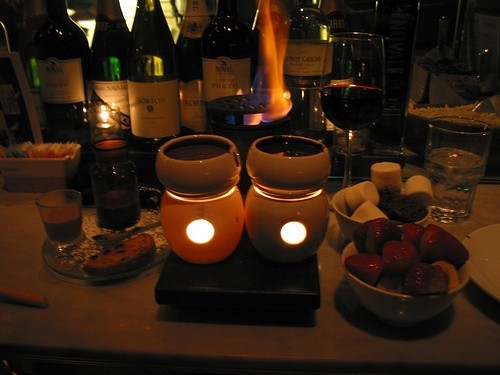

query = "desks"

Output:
[[0, 184, 500, 375]]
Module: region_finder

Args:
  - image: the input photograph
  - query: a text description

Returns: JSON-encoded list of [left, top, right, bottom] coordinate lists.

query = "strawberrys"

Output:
[[343, 217, 469, 296]]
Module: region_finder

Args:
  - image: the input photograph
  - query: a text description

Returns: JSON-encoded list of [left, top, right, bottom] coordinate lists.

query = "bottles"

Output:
[[278, 0, 356, 137], [18, 0, 91, 129], [84, 0, 132, 140], [89, 138, 142, 231], [175, 0, 215, 137], [128, 0, 182, 146], [202, 0, 256, 108]]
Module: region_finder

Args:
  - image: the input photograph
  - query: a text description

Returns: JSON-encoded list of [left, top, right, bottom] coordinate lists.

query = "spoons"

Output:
[[92, 220, 164, 243]]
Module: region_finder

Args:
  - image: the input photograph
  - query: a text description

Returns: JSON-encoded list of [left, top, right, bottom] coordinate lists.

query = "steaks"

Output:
[[82, 233, 154, 277]]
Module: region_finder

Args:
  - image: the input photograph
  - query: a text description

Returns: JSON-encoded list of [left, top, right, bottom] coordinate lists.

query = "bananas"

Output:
[[344, 162, 432, 223]]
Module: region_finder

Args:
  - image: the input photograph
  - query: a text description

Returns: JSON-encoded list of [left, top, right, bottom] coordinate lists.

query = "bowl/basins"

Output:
[[340, 239, 468, 328], [332, 185, 432, 243]]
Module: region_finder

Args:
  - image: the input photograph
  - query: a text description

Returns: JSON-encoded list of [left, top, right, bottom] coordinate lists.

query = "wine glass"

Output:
[[319, 32, 386, 212]]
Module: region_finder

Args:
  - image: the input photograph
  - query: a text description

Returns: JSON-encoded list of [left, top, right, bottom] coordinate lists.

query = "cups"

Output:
[[422, 115, 495, 225], [34, 189, 83, 247]]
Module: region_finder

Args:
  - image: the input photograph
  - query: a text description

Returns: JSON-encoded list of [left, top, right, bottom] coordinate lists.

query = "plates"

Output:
[[461, 223, 500, 304], [41, 209, 172, 283]]
